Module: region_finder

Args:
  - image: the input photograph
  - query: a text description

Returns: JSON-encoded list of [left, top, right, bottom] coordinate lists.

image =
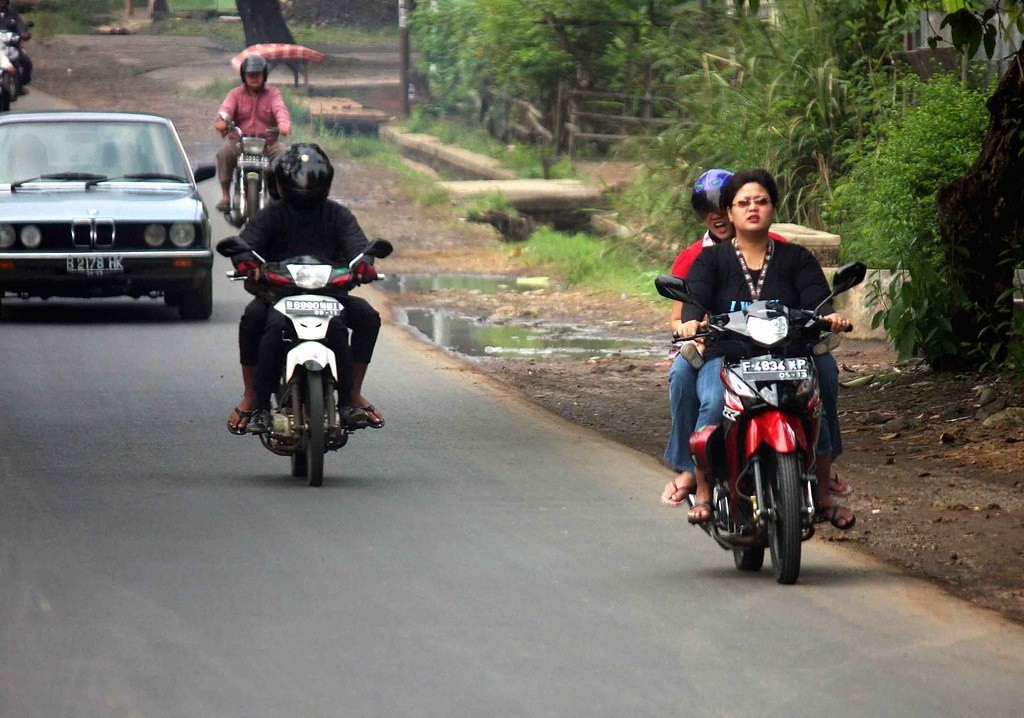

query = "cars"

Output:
[[0, 112, 217, 324]]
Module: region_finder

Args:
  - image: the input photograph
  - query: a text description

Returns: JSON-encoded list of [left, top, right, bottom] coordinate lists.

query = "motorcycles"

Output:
[[219, 109, 283, 228], [0, 19, 35, 112], [215, 234, 394, 487], [654, 262, 868, 585]]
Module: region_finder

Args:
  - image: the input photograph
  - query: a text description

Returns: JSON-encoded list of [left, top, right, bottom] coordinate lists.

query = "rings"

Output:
[[840, 320, 844, 322]]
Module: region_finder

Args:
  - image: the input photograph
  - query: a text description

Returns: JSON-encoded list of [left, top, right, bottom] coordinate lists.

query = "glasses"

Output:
[[732, 197, 771, 208]]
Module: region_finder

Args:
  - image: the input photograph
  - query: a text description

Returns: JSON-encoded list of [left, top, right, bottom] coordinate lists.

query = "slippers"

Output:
[[227, 407, 252, 435], [661, 479, 690, 507], [687, 501, 713, 523], [815, 500, 856, 529], [829, 472, 854, 498], [337, 401, 386, 429]]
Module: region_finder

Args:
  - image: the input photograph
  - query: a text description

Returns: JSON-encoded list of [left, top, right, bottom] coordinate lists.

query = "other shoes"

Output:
[[680, 342, 704, 370], [807, 333, 840, 356], [216, 200, 230, 211]]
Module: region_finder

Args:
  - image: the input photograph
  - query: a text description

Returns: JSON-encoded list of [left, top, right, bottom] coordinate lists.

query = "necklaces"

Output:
[[757, 259, 763, 268]]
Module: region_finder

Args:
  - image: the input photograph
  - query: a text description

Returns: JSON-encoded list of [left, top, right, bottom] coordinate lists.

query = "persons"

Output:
[[0, 0, 33, 93], [215, 55, 291, 211], [228, 143, 385, 435], [663, 168, 856, 533]]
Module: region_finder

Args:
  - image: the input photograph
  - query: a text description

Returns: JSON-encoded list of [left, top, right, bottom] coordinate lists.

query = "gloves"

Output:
[[353, 261, 377, 287], [235, 260, 257, 275]]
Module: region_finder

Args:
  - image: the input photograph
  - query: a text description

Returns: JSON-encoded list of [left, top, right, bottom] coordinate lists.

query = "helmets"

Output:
[[240, 57, 269, 87], [274, 143, 334, 209], [264, 154, 282, 200], [691, 169, 735, 219]]
